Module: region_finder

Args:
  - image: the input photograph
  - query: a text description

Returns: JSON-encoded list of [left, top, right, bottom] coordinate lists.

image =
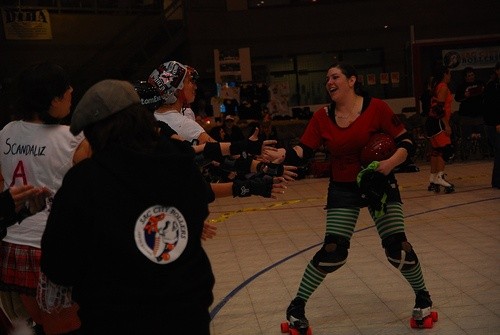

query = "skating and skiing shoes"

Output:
[[410, 291, 438, 328], [427, 172, 454, 194], [281, 299, 312, 335]]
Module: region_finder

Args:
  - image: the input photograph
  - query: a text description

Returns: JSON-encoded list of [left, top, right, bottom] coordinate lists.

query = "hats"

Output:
[[70, 79, 140, 136], [148, 60, 187, 104], [184, 65, 198, 76]]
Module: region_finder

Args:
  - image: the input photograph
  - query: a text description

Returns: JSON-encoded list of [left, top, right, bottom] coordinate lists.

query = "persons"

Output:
[[0, 70, 216, 335], [427, 64, 500, 194], [206, 116, 500, 182], [138, 60, 298, 201], [0, 186, 54, 239], [270, 58, 438, 335], [39, 80, 217, 335]]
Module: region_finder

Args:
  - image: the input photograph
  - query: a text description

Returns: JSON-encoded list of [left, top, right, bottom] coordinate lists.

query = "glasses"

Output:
[[186, 74, 199, 80]]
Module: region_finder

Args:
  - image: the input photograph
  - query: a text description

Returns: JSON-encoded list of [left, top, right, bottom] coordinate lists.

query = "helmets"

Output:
[[359, 133, 398, 168]]
